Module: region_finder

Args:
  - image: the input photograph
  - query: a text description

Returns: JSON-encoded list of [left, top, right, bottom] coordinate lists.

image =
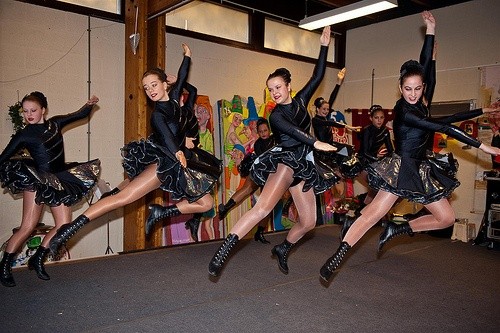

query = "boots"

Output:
[[403, 206, 432, 237], [145, 203, 182, 241], [320, 240, 352, 283], [254, 225, 270, 244], [184, 211, 203, 241], [99, 186, 121, 200], [208, 232, 239, 277], [339, 214, 362, 241], [378, 219, 414, 253], [0, 250, 20, 287], [282, 195, 293, 217], [50, 213, 91, 256], [271, 238, 296, 274], [354, 202, 367, 218], [218, 197, 236, 220], [28, 245, 51, 280]]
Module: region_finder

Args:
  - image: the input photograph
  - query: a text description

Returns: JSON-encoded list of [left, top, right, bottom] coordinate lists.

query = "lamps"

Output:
[[298, 0, 398, 31]]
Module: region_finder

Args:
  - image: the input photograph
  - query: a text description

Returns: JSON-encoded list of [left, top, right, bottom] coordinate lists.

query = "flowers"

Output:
[[327, 197, 361, 217]]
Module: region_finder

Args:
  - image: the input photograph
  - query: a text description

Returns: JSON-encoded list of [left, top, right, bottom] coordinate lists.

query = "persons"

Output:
[[318, 9, 500, 284], [217, 116, 280, 245], [338, 102, 397, 241], [0, 87, 104, 291], [205, 24, 344, 278], [49, 41, 225, 262], [310, 66, 361, 196]]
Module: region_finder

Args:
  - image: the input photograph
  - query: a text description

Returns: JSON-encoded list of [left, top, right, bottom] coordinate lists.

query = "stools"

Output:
[[451, 223, 476, 243]]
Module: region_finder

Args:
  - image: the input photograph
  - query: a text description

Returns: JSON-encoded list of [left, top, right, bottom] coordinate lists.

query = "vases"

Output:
[[333, 213, 346, 225]]
